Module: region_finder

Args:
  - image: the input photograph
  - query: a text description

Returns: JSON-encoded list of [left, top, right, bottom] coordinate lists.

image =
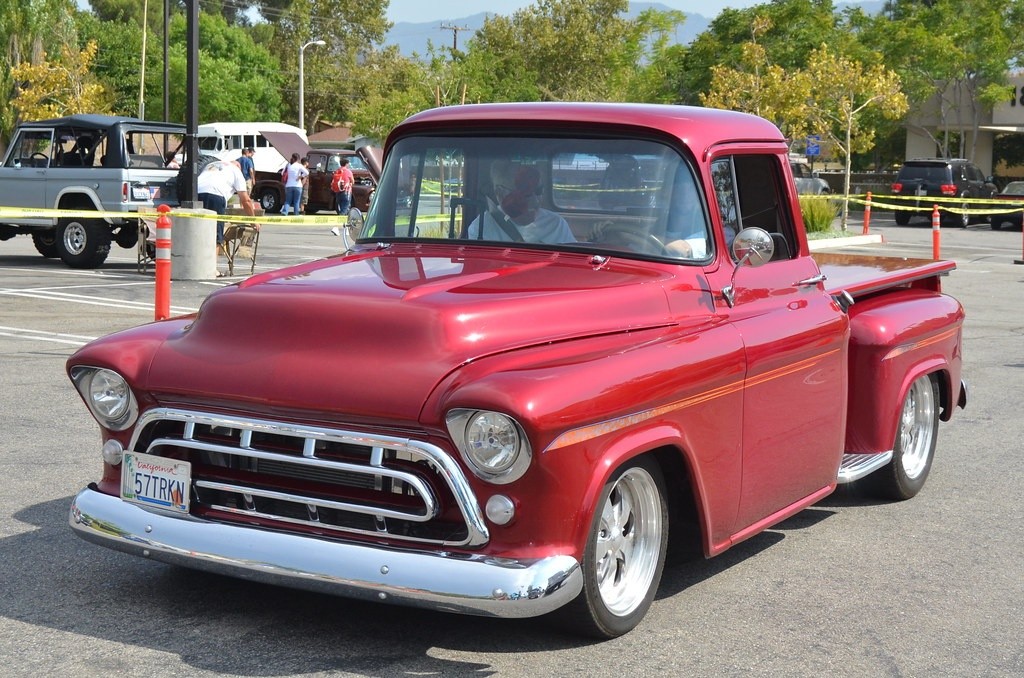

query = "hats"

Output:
[[248, 148, 255, 154]]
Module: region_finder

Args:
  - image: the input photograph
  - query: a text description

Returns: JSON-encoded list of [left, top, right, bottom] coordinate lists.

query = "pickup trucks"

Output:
[[65, 103, 968, 643], [250, 131, 414, 216]]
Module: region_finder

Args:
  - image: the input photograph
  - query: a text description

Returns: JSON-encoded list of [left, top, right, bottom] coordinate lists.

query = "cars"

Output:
[[991, 182, 1024, 229]]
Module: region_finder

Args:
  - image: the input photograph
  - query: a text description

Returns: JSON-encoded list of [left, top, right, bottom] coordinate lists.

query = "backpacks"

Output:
[[330, 168, 350, 192]]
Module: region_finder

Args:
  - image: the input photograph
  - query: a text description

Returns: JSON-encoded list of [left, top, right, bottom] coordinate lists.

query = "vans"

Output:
[[175, 122, 309, 174]]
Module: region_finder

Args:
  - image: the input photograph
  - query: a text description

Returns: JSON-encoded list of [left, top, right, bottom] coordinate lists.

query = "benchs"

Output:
[[130, 154, 164, 169], [554, 211, 658, 244]]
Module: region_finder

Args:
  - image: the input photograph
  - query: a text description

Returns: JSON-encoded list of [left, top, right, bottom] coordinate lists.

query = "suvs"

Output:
[[0, 115, 221, 270], [892, 157, 998, 228], [789, 161, 831, 196]]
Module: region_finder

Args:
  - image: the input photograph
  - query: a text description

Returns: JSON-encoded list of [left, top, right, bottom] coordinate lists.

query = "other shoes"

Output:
[[216, 272, 227, 277], [331, 227, 340, 237]]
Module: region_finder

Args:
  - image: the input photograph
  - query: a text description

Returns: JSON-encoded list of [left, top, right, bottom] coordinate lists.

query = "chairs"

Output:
[[219, 223, 260, 277], [54, 152, 90, 168], [137, 206, 158, 274]]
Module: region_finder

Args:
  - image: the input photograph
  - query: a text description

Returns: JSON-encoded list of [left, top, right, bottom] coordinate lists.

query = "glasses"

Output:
[[496, 183, 544, 196]]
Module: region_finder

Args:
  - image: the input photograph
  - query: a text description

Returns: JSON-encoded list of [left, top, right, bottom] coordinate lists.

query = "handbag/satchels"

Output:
[[282, 163, 289, 183]]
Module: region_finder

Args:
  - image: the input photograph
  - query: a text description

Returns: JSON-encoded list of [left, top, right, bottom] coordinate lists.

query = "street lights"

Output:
[[299, 40, 326, 133]]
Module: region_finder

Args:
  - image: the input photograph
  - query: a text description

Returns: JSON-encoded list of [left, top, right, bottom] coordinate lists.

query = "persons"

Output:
[[300, 158, 309, 215], [331, 158, 354, 236], [467, 160, 576, 244], [163, 151, 179, 168], [198, 161, 260, 277], [589, 159, 736, 259], [236, 147, 256, 207], [281, 153, 309, 216]]
